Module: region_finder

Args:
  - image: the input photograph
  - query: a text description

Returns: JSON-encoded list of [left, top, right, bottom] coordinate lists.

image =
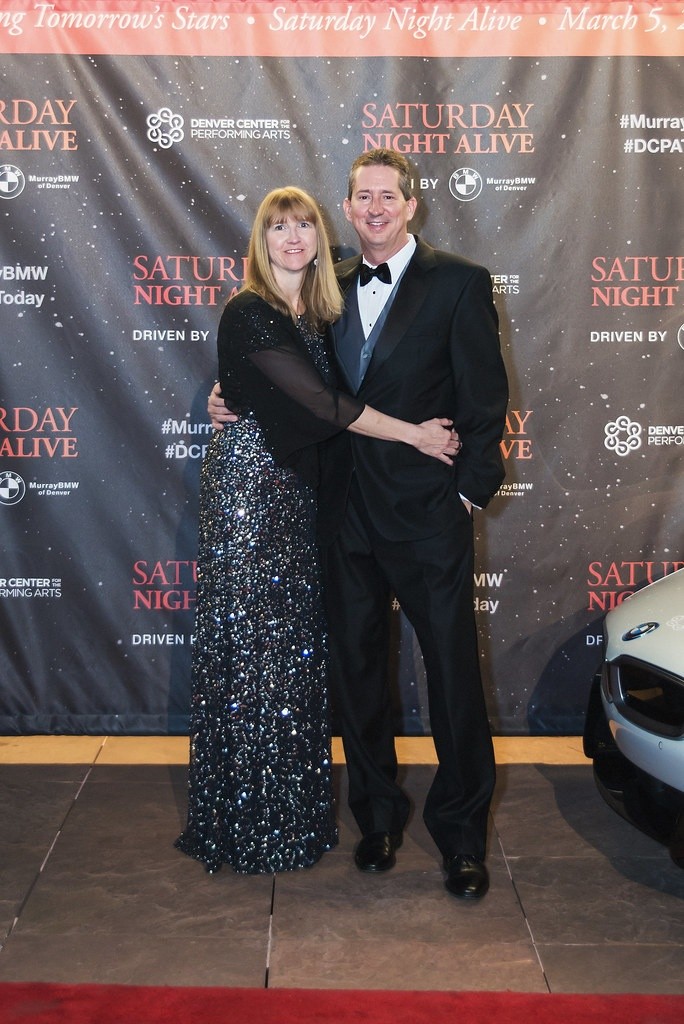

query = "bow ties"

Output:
[[360, 263, 392, 287]]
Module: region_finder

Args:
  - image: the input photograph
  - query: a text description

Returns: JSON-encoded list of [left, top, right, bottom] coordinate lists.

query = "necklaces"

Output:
[[291, 300, 300, 319]]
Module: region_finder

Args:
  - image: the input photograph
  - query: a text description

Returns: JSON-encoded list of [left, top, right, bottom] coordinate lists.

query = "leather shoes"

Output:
[[355, 831, 403, 872], [443, 854, 489, 900]]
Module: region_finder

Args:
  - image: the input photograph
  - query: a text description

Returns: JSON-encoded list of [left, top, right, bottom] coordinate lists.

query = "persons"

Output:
[[173, 149, 509, 898]]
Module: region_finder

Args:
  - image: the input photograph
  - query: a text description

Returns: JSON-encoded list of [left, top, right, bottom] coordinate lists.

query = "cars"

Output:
[[582, 567, 684, 868]]
